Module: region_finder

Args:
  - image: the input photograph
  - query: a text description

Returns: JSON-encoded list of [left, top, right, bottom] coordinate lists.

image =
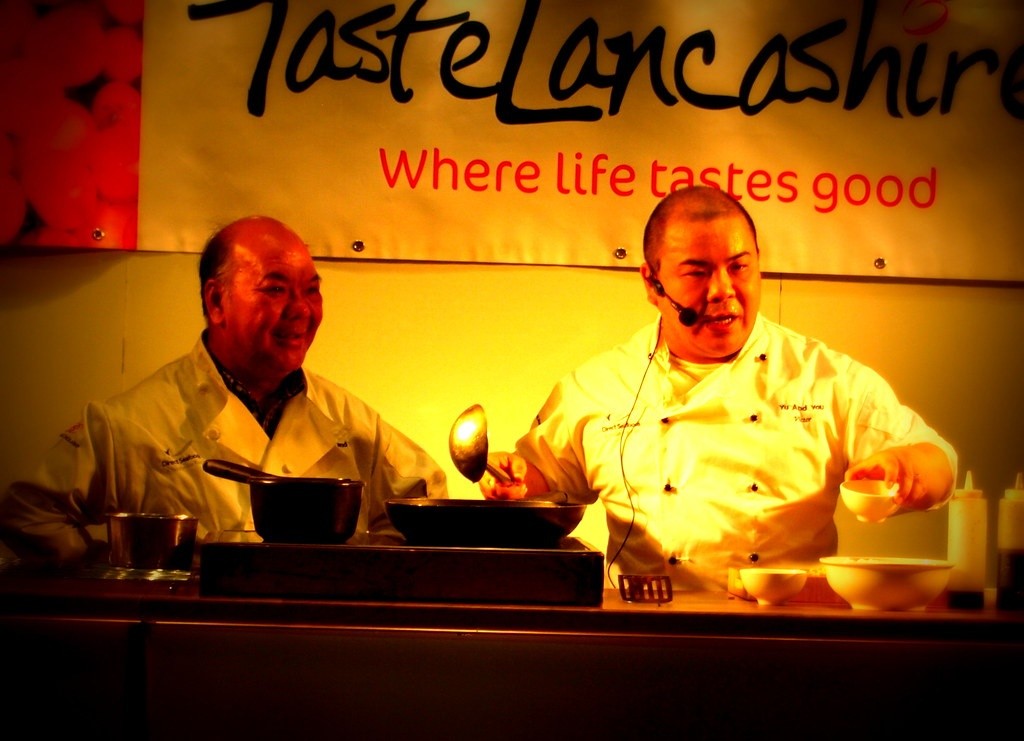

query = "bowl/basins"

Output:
[[104, 512, 198, 571], [739, 568, 807, 605]]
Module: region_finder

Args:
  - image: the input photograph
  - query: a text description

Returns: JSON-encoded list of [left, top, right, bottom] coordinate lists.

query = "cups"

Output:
[[984, 588, 996, 610]]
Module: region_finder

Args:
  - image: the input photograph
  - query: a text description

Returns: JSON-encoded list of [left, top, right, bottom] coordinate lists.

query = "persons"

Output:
[[0, 214, 425, 578], [480, 185, 959, 591]]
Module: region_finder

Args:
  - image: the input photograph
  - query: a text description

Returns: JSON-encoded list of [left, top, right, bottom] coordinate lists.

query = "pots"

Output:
[[840, 480, 900, 524], [384, 492, 586, 549], [819, 556, 954, 612], [202, 459, 363, 544]]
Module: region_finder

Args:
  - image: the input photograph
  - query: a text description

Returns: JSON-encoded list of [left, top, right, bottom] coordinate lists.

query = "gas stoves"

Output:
[[199, 528, 605, 606]]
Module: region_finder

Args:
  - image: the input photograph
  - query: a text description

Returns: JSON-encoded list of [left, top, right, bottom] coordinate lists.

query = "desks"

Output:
[[0, 557, 1024, 741]]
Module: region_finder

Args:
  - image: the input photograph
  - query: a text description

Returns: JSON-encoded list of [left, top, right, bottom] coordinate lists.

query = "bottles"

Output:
[[948, 470, 986, 611], [996, 473, 1024, 611]]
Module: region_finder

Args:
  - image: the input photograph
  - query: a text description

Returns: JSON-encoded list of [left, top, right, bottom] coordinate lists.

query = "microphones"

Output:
[[653, 278, 698, 327]]
